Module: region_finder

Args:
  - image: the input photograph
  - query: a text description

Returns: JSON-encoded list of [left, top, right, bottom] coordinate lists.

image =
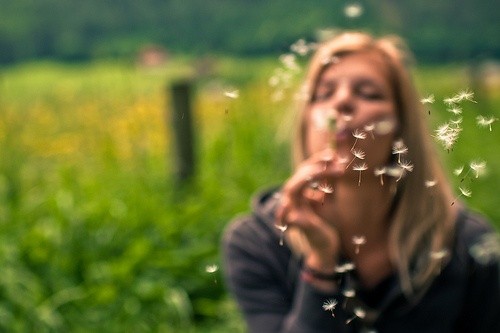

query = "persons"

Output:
[[219, 32, 500, 333]]
